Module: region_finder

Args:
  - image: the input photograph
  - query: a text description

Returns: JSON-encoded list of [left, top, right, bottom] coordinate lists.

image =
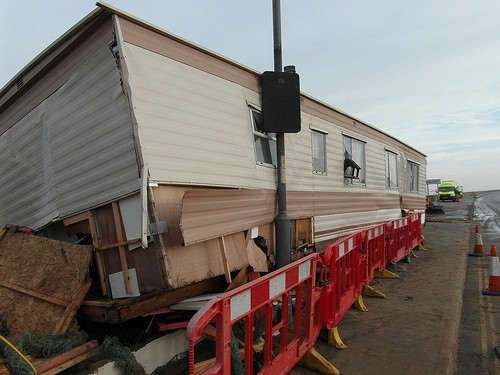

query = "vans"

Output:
[[435, 181, 463, 202]]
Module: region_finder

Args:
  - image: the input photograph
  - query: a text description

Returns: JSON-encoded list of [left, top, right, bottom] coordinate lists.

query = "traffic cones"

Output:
[[482, 243, 500, 296], [468, 225, 490, 256]]
